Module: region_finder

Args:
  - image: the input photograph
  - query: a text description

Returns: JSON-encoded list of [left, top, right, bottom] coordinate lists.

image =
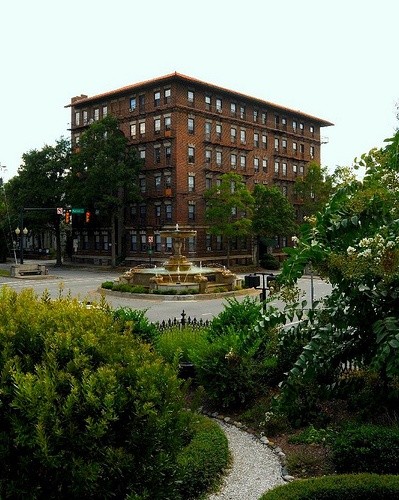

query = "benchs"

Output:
[[11, 264, 46, 275]]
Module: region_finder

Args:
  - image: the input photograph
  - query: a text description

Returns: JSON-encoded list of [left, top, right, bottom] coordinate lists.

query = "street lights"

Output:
[[290, 235, 315, 311], [15, 224, 28, 263], [244, 272, 281, 316]]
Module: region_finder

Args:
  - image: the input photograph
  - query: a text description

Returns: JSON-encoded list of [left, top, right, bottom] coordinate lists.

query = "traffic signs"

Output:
[[71, 208, 85, 213]]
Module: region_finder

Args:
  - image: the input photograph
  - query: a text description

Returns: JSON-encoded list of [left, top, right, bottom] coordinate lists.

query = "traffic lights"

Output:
[[65, 212, 71, 224], [86, 211, 91, 223]]
